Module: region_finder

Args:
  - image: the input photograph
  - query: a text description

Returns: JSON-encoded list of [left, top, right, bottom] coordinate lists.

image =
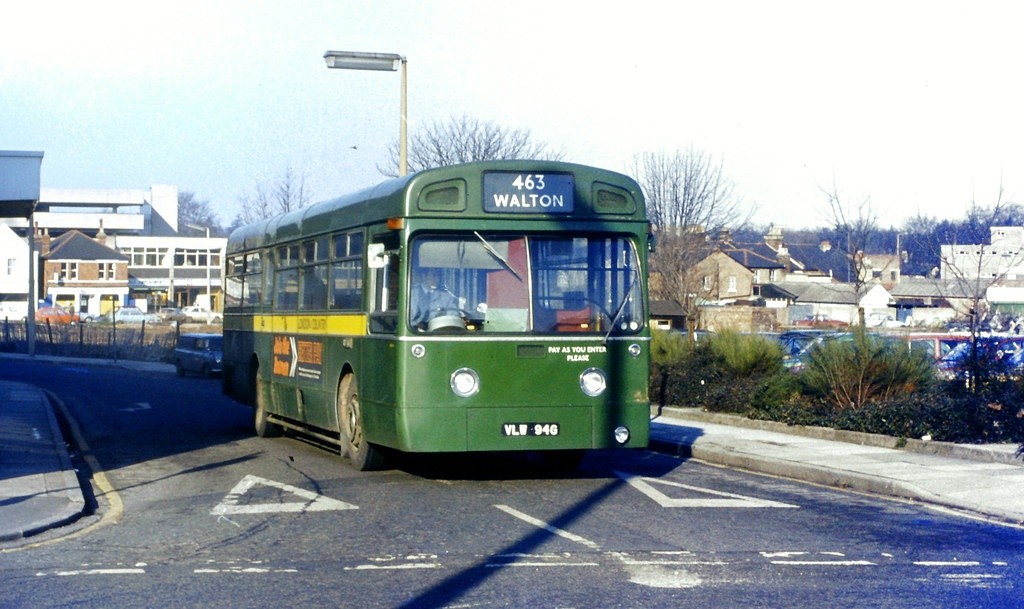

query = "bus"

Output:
[[222, 166, 655, 469]]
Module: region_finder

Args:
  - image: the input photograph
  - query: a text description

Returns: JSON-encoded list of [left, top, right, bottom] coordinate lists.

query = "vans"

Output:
[[0, 301, 28, 323], [174, 332, 228, 379]]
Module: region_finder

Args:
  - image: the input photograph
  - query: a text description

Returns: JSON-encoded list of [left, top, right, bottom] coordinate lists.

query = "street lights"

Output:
[[326, 49, 416, 181]]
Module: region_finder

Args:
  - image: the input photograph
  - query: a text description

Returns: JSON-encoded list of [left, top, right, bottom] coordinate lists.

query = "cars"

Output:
[[183, 306, 224, 324], [157, 307, 193, 325], [108, 306, 164, 324], [669, 325, 1023, 390], [37, 307, 81, 326], [77, 311, 98, 323]]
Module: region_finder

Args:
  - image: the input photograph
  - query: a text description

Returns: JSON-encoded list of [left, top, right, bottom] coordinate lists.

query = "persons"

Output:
[[410, 267, 486, 329]]
